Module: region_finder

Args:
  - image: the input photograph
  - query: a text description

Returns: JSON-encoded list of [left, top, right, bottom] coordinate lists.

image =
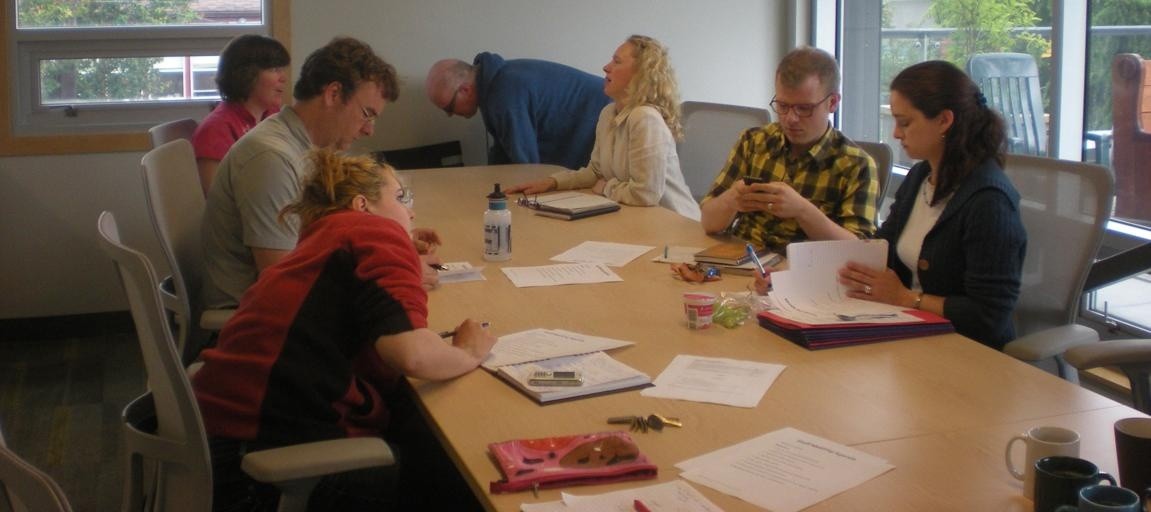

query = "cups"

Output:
[[1052, 480, 1141, 512], [1028, 454, 1117, 512], [1114, 417, 1151, 501], [682, 292, 716, 331], [1003, 426, 1083, 502]]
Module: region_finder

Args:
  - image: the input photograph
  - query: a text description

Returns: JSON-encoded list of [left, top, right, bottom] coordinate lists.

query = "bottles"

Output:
[[482, 183, 513, 262]]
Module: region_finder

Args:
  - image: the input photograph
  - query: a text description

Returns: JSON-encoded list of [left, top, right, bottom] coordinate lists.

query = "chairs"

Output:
[[367, 138, 465, 172], [95, 119, 395, 509], [679, 101, 771, 206], [856, 58, 1151, 409], [0, 437, 74, 512]]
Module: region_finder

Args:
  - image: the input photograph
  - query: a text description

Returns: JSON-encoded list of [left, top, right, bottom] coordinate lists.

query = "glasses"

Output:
[[442, 90, 457, 114], [769, 92, 835, 119], [345, 84, 380, 121]]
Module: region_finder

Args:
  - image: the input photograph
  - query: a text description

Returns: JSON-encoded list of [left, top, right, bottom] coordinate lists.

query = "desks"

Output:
[[353, 163, 1151, 512]]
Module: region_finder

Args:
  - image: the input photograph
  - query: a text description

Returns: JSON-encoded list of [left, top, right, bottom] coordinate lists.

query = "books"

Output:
[[479, 329, 656, 405], [516, 192, 620, 220], [652, 241, 782, 276]]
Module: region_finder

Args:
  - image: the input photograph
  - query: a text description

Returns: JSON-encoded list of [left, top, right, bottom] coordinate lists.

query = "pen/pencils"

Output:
[[746, 244, 775, 292], [439, 323, 489, 338], [665, 246, 668, 258], [430, 265, 448, 271]]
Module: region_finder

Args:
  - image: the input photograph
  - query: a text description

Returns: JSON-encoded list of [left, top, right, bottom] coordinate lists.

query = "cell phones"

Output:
[[528, 369, 583, 386], [743, 174, 764, 185]]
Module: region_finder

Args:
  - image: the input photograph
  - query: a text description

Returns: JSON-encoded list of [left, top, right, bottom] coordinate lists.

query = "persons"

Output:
[[198, 35, 442, 349], [501, 34, 703, 222], [191, 145, 496, 511], [755, 60, 1028, 351], [428, 51, 616, 171], [192, 36, 290, 200], [699, 46, 881, 259]]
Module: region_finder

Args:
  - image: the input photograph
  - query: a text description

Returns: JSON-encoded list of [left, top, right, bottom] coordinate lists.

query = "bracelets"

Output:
[[913, 293, 923, 308]]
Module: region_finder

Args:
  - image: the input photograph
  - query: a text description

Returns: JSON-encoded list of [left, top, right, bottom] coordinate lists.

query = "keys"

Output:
[[608, 413, 683, 434]]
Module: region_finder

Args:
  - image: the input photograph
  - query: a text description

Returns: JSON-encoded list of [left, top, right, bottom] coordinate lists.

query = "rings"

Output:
[[768, 203, 773, 209], [864, 286, 871, 293]]
